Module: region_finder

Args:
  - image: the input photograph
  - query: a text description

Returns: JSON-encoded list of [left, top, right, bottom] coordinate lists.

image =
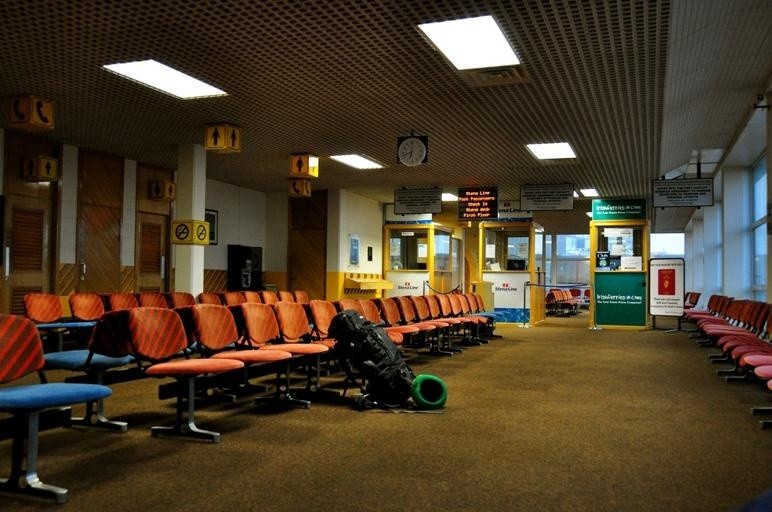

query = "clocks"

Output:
[[395, 129, 431, 167]]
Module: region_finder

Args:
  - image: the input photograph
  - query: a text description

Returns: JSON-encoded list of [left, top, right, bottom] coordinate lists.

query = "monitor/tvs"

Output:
[[507, 259, 525, 270]]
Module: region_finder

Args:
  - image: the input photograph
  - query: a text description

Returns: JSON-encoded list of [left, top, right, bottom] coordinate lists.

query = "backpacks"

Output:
[[329, 310, 415, 409]]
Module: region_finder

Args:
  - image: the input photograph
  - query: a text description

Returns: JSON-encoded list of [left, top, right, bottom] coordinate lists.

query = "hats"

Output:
[[411, 374, 447, 409]]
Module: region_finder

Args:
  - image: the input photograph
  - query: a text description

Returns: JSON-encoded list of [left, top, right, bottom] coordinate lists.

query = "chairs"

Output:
[[543, 281, 592, 321], [1, 313, 113, 506], [24, 290, 510, 444], [678, 288, 772, 434]]
